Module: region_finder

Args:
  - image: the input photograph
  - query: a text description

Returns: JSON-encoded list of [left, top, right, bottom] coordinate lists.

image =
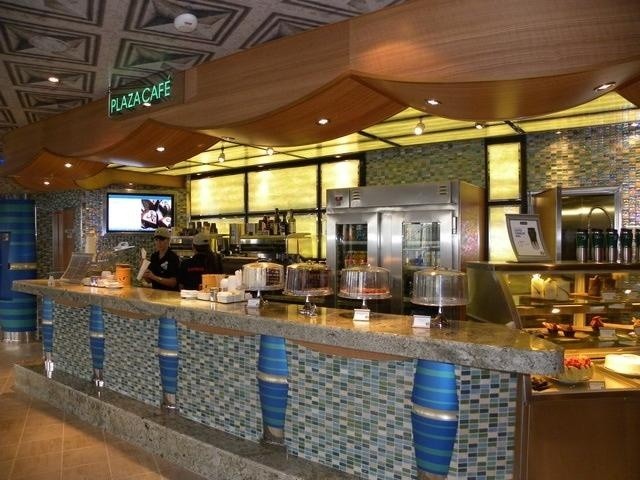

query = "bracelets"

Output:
[[156, 277, 162, 283]]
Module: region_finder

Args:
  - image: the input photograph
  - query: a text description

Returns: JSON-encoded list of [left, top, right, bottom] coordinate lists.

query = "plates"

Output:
[[519, 295, 574, 302], [568, 293, 602, 301], [544, 375, 590, 384], [179, 289, 210, 300], [106, 285, 124, 289], [542, 335, 578, 342]]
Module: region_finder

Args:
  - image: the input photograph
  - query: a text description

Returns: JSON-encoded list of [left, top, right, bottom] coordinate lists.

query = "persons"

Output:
[[142, 227, 181, 292], [186, 231, 223, 290]]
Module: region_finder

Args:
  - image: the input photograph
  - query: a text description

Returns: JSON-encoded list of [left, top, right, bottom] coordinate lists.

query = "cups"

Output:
[[177, 228, 198, 236]]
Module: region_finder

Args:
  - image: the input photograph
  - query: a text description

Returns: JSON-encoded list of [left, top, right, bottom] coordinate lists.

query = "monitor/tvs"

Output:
[[106, 192, 175, 233]]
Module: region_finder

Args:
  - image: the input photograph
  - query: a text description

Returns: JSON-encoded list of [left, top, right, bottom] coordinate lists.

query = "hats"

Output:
[[193, 233, 210, 245], [154, 228, 170, 238]]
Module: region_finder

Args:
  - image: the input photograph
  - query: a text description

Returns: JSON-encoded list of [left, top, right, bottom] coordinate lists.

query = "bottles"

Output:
[[344, 248, 367, 270], [219, 279, 228, 292], [406, 274, 413, 297], [575, 228, 640, 263], [210, 287, 219, 302], [274, 251, 303, 266], [339, 223, 367, 242], [262, 207, 297, 236]]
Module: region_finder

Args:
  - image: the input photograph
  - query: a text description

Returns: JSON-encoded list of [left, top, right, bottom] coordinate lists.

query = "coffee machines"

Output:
[[217, 234, 287, 274]]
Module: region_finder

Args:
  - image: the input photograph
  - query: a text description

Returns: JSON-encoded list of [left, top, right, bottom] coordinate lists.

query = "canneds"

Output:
[[575, 230, 640, 265]]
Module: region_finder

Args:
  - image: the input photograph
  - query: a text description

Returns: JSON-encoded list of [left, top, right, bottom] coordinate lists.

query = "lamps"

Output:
[[411, 107, 488, 135], [162, 145, 276, 171]]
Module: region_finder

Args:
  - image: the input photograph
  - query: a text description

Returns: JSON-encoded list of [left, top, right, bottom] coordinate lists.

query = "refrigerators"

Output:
[[326, 179, 488, 316]]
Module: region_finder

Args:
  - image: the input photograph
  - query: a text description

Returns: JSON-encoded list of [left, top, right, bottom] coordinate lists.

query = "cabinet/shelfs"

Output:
[[224, 231, 314, 268], [467, 260, 640, 404], [167, 235, 228, 260]]
